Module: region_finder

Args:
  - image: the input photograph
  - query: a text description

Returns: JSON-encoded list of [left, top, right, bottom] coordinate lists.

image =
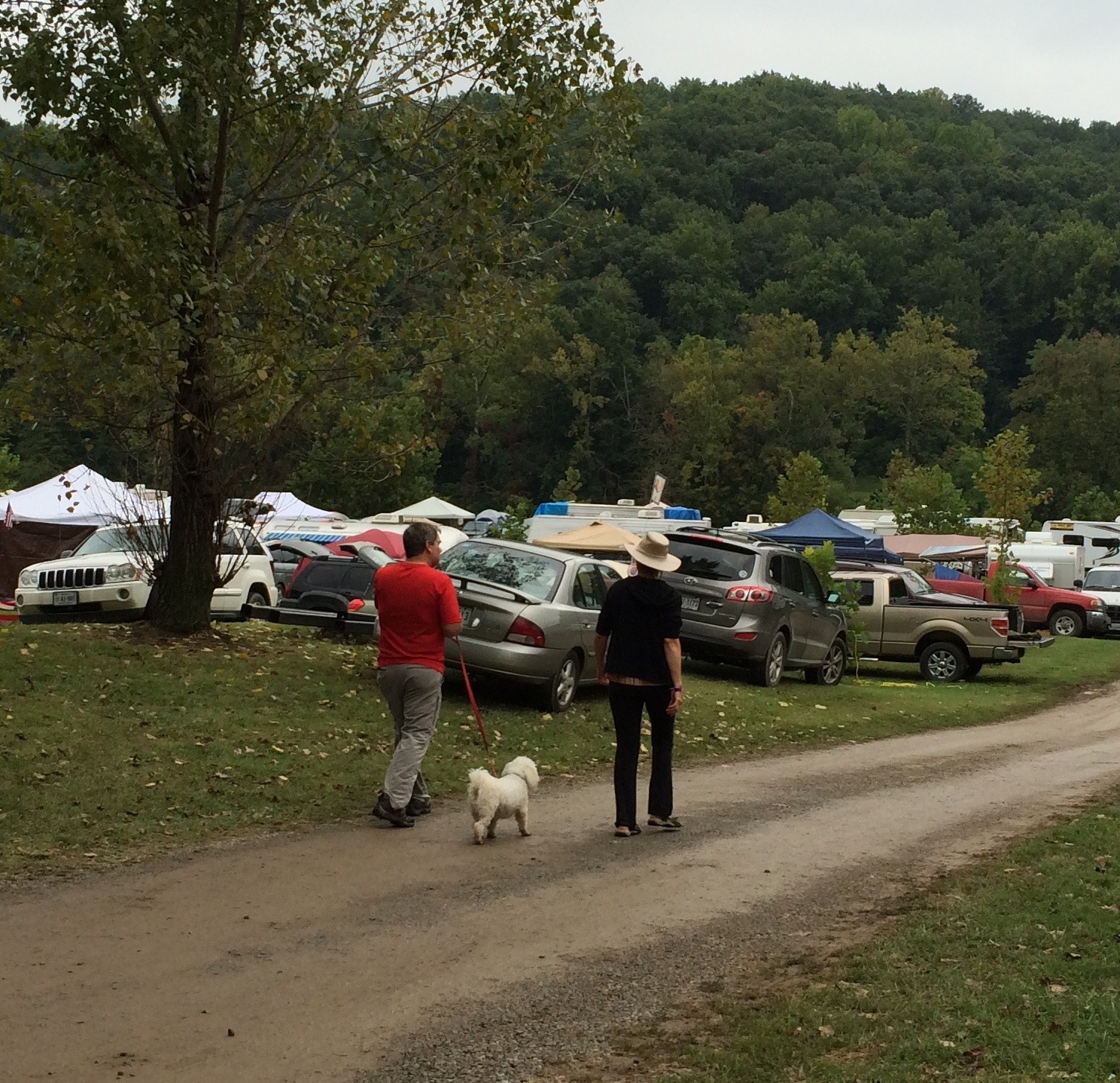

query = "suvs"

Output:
[[657, 525, 849, 689], [15, 516, 278, 624]]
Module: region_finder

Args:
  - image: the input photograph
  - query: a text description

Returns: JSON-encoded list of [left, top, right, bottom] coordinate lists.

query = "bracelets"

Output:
[[673, 687, 682, 692]]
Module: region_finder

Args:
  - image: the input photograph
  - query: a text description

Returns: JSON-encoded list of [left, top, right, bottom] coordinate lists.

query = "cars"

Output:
[[373, 535, 624, 712]]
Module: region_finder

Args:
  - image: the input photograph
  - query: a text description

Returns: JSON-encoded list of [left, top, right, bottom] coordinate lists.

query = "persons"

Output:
[[372, 522, 463, 827], [594, 531, 684, 837]]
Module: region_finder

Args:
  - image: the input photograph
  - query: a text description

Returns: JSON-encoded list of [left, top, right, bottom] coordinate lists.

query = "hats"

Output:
[[624, 532, 681, 572]]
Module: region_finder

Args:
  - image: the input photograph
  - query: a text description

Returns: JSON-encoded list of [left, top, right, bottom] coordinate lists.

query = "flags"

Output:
[[4, 502, 14, 528]]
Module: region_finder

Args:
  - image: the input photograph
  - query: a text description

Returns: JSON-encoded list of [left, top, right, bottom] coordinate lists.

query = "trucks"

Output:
[[253, 497, 1120, 616]]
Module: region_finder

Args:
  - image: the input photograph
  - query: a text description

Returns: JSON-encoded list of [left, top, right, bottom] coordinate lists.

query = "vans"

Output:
[[1073, 565, 1120, 635]]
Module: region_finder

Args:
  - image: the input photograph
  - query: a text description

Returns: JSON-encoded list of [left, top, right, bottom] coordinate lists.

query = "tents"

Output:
[[0, 465, 166, 601], [748, 508, 904, 566], [157, 492, 475, 591], [533, 522, 641, 563]]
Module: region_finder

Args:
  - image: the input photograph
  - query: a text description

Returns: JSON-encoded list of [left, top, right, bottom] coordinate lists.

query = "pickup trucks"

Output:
[[819, 570, 1055, 683], [923, 560, 1112, 638]]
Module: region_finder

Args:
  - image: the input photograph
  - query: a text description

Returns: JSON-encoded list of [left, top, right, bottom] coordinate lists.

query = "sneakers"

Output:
[[648, 814, 683, 828], [616, 824, 642, 836], [372, 792, 415, 827], [407, 799, 432, 815]]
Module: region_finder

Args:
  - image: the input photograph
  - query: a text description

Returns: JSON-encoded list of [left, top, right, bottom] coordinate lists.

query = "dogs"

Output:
[[467, 755, 541, 845]]
